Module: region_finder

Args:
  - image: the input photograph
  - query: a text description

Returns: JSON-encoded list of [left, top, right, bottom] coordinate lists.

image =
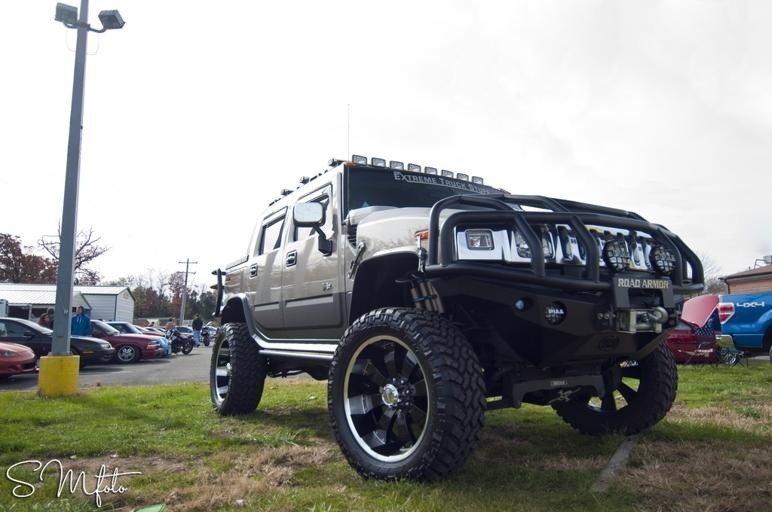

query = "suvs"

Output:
[[208, 153, 706, 483]]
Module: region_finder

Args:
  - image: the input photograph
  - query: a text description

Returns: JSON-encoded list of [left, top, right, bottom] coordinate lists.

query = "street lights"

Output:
[[46, 0, 127, 356]]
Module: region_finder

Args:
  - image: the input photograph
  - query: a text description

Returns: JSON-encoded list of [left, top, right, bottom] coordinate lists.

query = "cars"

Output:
[[666, 292, 741, 367], [0, 315, 219, 383]]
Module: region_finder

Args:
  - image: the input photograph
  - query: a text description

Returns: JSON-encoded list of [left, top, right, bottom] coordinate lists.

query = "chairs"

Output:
[[678, 342, 717, 369], [714, 333, 750, 368]]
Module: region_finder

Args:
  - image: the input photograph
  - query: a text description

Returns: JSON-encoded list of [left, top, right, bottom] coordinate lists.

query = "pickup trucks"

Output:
[[719, 291, 771, 362]]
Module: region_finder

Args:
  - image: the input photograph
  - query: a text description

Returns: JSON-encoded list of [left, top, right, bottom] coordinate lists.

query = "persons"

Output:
[[147, 321, 155, 328], [192, 314, 203, 347], [37, 313, 50, 327], [165, 316, 175, 330], [70, 305, 91, 335], [47, 308, 54, 329]]
